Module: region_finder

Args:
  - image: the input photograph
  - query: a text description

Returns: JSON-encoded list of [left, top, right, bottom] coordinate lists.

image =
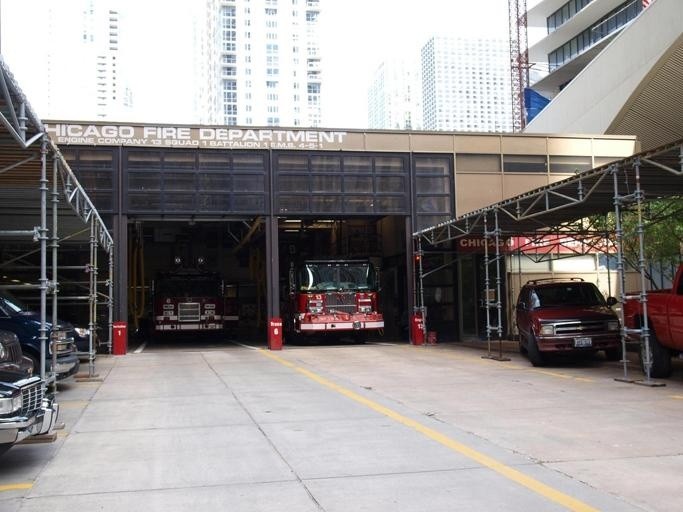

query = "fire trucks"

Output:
[[280, 259, 384, 343], [151, 256, 239, 336]]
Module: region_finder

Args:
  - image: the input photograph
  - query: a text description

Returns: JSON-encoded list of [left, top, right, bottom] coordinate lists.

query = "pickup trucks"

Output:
[[622, 264, 682, 378]]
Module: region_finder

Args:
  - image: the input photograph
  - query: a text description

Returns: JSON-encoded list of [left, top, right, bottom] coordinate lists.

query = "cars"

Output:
[[517, 278, 621, 366], [0, 293, 97, 458]]
